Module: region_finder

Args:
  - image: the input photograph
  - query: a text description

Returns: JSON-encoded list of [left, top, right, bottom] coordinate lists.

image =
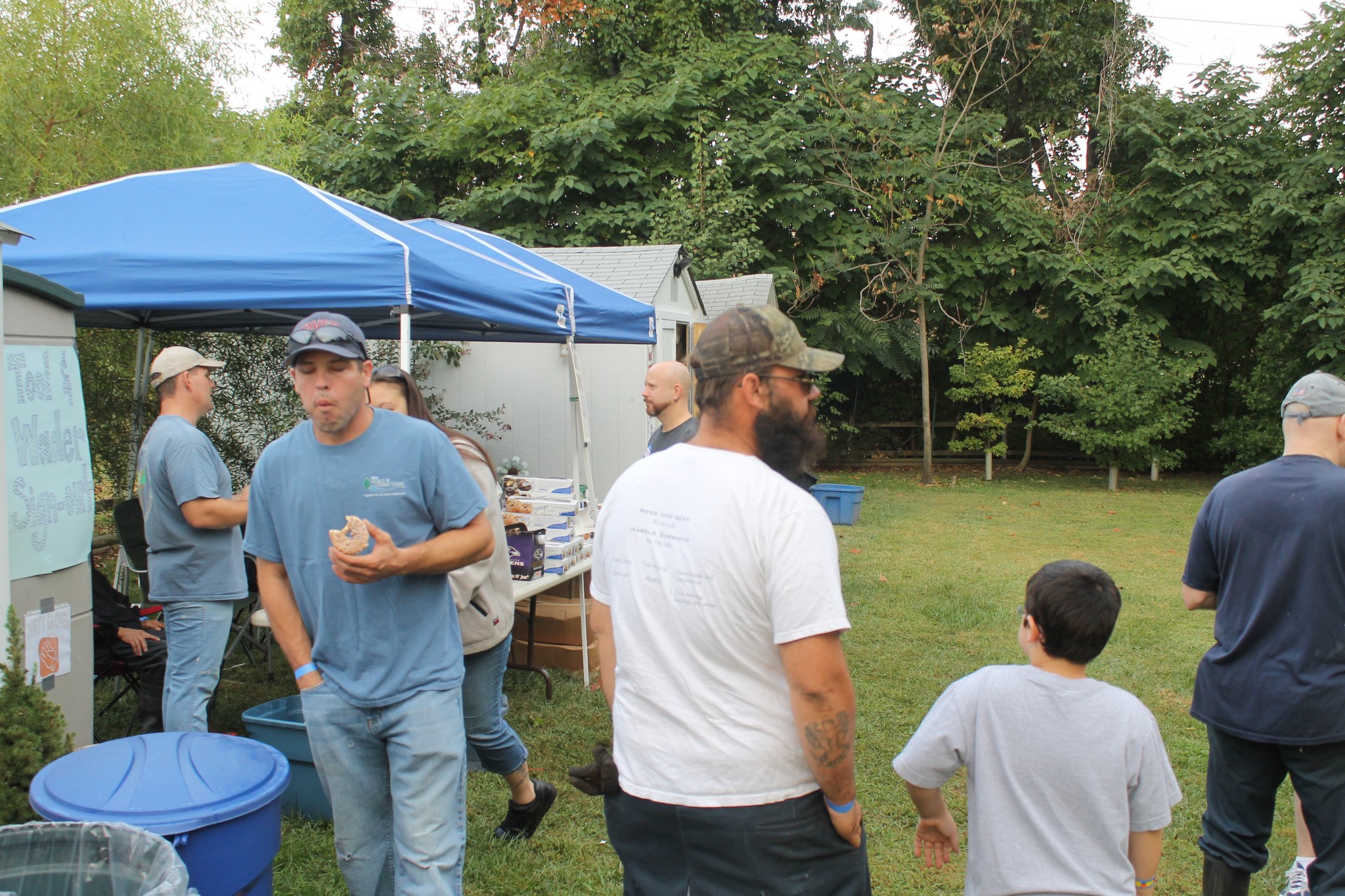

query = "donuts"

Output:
[[329, 515, 370, 554], [503, 478, 533, 533]]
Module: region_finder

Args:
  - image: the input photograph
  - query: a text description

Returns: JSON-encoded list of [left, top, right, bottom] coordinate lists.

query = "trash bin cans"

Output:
[[0, 821, 190, 896], [28, 730, 291, 896]]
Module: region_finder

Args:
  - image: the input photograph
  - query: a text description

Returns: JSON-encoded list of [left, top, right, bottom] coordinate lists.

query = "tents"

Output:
[[0, 161, 657, 691]]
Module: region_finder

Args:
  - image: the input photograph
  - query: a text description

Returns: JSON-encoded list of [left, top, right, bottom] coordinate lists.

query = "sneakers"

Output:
[[1278, 857, 1318, 896], [494, 778, 557, 838]]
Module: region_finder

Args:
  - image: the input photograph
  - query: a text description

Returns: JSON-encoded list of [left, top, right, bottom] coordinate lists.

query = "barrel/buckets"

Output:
[[29, 728, 291, 896]]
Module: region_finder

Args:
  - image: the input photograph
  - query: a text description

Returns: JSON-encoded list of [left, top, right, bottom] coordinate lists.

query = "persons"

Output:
[[590, 305, 870, 896], [1278, 790, 1316, 896], [136, 347, 251, 733], [362, 365, 558, 841], [642, 361, 700, 458], [242, 311, 496, 896], [1182, 370, 1345, 896], [892, 560, 1182, 896], [91, 552, 167, 735]]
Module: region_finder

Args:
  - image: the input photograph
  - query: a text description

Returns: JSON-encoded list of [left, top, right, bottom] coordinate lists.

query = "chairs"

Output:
[[114, 499, 149, 609], [220, 554, 276, 682], [84, 606, 162, 746]]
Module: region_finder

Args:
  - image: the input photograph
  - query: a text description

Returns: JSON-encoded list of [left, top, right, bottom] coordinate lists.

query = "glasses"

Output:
[[288, 327, 368, 359], [758, 375, 816, 394], [373, 365, 406, 381]]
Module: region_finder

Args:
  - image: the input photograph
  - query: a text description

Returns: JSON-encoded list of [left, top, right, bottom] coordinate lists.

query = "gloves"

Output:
[[569, 745, 620, 799]]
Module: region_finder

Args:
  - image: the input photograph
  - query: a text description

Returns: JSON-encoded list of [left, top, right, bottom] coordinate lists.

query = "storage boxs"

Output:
[[809, 483, 865, 527], [242, 694, 335, 826], [502, 476, 606, 580], [508, 594, 603, 673]]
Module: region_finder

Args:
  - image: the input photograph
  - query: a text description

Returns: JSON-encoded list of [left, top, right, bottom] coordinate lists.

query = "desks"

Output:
[[510, 558, 595, 702]]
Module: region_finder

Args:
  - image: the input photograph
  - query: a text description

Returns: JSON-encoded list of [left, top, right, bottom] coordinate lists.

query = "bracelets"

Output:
[[825, 796, 854, 813], [294, 662, 319, 679]]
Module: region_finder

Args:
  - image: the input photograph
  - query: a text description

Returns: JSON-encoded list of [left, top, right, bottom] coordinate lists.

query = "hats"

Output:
[[151, 346, 227, 389], [1281, 371, 1345, 424], [690, 304, 845, 379], [282, 311, 366, 367]]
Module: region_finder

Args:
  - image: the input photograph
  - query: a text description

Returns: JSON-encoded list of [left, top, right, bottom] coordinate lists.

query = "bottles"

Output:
[[580, 489, 598, 519]]
